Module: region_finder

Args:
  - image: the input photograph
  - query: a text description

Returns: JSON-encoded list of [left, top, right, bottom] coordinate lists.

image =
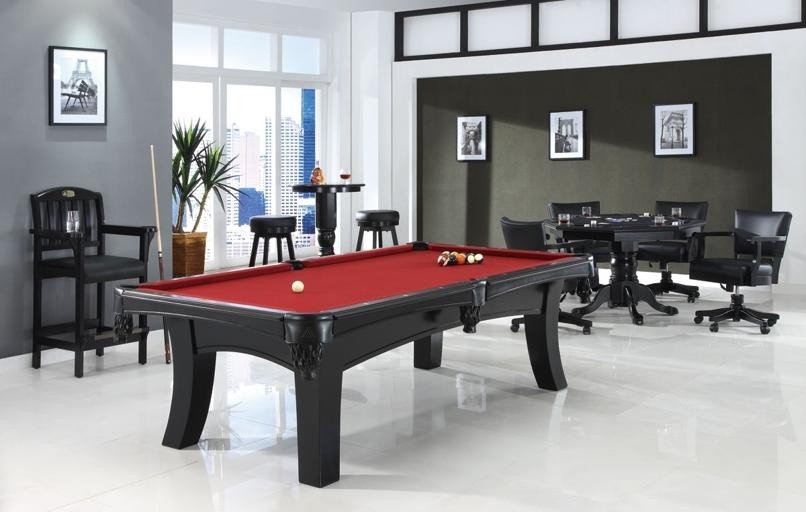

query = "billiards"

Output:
[[438, 251, 485, 266], [292, 281, 304, 293]]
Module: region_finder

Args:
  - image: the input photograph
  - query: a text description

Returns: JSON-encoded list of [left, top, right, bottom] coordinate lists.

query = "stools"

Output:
[[355, 210, 400, 251], [249, 214, 297, 266]]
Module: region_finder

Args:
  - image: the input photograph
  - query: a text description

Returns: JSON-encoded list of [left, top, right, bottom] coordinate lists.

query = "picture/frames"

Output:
[[650, 101, 697, 157], [548, 108, 587, 161], [455, 113, 488, 162], [49, 45, 106, 126]]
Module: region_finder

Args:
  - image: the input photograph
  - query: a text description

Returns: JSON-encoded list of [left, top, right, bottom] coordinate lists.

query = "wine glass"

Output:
[[339, 168, 351, 184]]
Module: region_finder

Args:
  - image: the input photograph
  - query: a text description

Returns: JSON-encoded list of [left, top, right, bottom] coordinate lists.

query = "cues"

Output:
[[150, 144, 171, 364]]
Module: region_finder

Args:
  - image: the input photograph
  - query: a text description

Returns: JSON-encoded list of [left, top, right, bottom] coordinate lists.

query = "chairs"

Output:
[[548, 200, 611, 303], [637, 201, 710, 302], [688, 209, 793, 334], [28, 186, 158, 378], [500, 216, 595, 335]]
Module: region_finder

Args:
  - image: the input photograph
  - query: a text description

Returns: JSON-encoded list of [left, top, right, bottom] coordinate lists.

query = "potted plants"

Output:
[[172, 118, 256, 277]]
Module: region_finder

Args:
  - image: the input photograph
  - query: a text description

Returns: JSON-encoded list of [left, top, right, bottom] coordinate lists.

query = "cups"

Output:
[[558, 205, 684, 227]]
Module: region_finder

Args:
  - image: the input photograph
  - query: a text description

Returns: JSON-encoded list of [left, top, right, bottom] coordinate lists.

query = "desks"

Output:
[[112, 240, 596, 488], [292, 183, 364, 258]]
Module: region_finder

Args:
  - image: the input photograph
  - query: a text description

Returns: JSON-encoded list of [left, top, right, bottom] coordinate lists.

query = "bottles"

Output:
[[310, 161, 325, 184]]
